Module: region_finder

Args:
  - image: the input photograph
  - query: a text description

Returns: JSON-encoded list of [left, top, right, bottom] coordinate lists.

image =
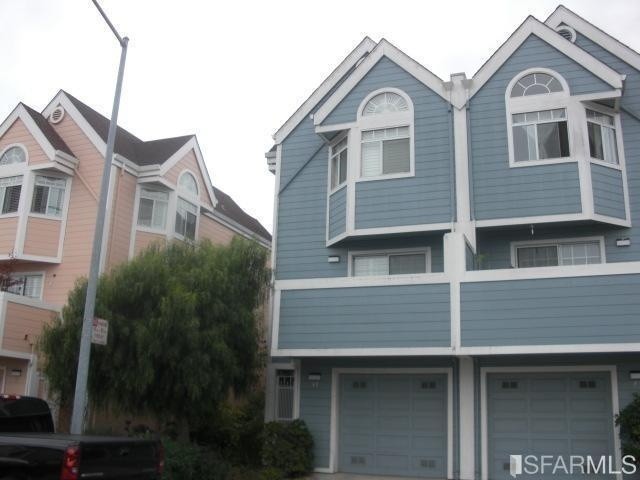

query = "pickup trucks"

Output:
[[0, 394, 164, 479]]
